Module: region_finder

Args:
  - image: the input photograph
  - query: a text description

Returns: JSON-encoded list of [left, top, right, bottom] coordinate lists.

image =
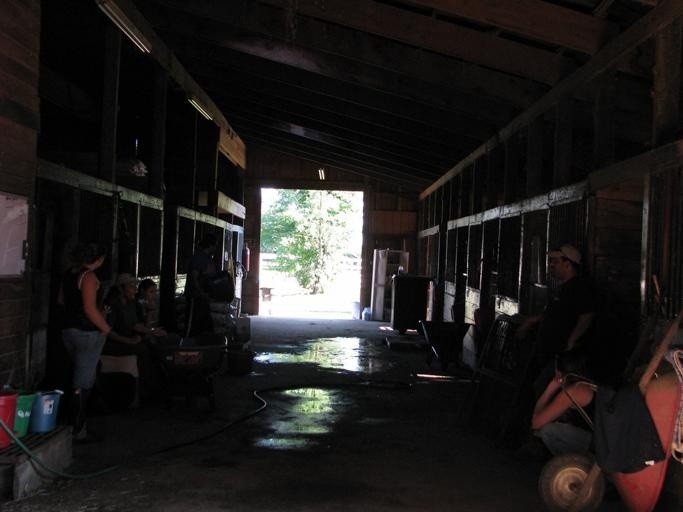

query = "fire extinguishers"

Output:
[[242, 242, 250, 272]]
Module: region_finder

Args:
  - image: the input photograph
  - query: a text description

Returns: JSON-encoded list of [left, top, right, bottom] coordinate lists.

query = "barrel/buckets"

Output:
[[226, 350, 254, 375], [0, 393, 16, 447], [12, 387, 38, 440], [29, 388, 65, 432]]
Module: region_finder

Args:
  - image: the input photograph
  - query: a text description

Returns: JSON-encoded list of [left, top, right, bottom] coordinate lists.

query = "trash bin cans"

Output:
[[390, 274, 436, 333]]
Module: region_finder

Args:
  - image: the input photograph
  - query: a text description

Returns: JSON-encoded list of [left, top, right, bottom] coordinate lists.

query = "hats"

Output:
[[546, 245, 581, 264], [118, 273, 139, 285]]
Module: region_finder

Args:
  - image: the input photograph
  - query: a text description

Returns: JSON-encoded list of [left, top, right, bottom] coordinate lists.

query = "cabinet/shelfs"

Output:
[[369, 249, 410, 322]]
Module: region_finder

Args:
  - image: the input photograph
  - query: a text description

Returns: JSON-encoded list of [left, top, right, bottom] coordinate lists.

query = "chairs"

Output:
[[145, 335, 218, 413]]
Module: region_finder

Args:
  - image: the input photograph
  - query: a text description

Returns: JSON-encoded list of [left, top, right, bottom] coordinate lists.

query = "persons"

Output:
[[55, 234, 112, 394], [516, 244, 595, 398], [106, 274, 164, 356], [529, 348, 597, 462]]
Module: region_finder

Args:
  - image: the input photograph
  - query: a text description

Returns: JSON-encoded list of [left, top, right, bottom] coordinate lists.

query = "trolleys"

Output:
[[539, 303, 682, 512]]
